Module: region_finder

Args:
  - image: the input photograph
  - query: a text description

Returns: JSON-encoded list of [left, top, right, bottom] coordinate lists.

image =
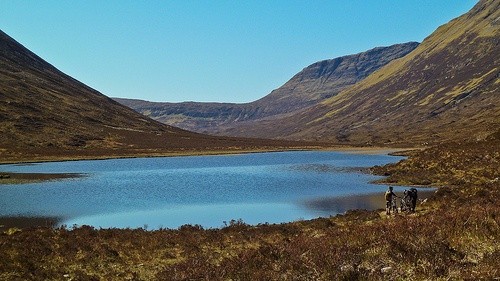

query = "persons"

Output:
[[384, 186, 398, 215], [403, 188, 418, 212]]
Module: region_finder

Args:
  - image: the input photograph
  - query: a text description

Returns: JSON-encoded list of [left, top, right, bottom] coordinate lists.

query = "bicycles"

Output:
[[391, 197, 398, 214], [400, 195, 412, 213]]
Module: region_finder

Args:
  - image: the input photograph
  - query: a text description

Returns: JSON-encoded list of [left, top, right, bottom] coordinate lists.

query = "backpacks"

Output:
[[385, 192, 392, 200], [410, 187, 417, 194]]
